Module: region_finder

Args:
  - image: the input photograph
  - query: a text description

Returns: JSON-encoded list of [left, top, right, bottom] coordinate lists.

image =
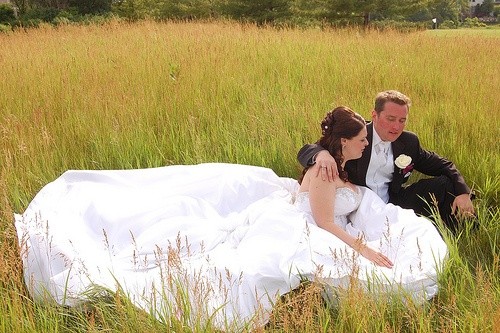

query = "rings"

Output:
[[321, 165, 326, 168]]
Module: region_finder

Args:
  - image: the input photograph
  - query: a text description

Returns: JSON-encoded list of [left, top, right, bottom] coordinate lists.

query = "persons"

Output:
[[288, 105, 450, 307], [296, 90, 480, 234]]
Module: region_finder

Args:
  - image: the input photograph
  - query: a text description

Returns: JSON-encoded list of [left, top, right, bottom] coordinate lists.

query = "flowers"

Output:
[[393, 154, 414, 175]]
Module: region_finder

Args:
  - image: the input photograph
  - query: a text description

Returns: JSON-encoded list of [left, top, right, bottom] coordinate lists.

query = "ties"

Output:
[[366, 144, 387, 195]]
